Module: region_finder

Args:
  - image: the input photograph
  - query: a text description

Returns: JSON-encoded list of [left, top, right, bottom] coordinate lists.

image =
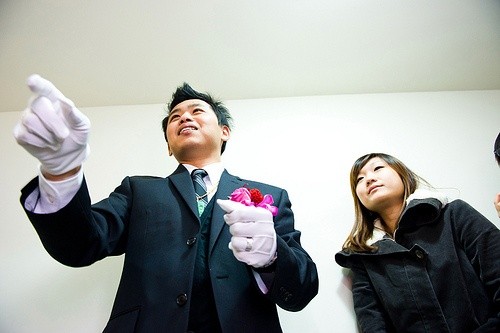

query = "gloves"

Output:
[[14, 74, 91, 176], [217, 198, 277, 269]]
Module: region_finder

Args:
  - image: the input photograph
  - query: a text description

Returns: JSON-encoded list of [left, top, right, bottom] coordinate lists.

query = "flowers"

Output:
[[230, 184, 279, 216]]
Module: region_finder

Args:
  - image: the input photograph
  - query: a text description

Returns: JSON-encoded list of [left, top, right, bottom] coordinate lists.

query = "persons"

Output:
[[333, 153, 500, 333], [13, 72, 320, 333], [493, 133, 500, 219]]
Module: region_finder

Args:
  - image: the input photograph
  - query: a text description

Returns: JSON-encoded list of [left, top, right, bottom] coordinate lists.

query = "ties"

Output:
[[191, 169, 208, 217]]
